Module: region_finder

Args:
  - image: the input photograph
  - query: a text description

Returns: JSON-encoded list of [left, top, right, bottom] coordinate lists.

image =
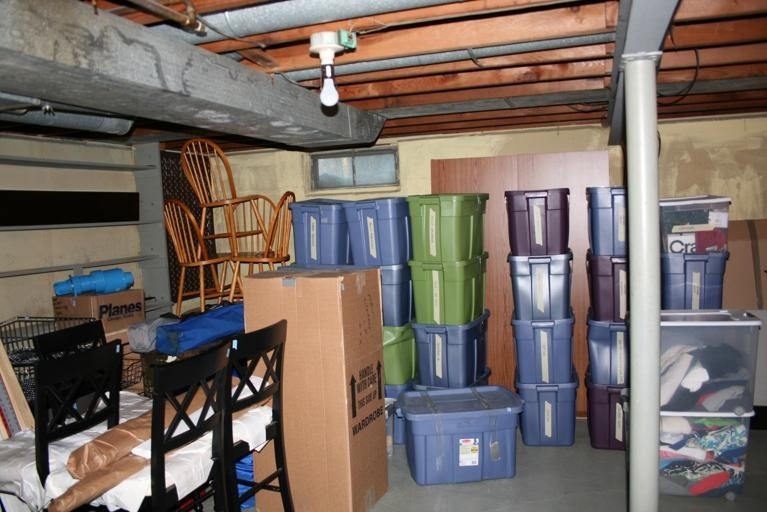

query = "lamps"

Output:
[[310, 30, 359, 107]]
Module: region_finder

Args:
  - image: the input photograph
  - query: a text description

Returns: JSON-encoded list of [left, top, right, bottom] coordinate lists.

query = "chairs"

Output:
[[218, 195, 275, 306], [161, 201, 247, 315], [75, 335, 234, 512], [224, 190, 295, 303], [181, 138, 275, 260], [190, 319, 297, 512], [1, 338, 124, 512]]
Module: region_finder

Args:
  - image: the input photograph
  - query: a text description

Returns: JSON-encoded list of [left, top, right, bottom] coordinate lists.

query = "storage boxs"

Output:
[[342, 197, 408, 266], [244, 270, 389, 512], [382, 323, 418, 385], [386, 397, 399, 459], [409, 250, 490, 326], [507, 254, 574, 321], [412, 367, 492, 392], [399, 385, 524, 486], [510, 319, 575, 384], [504, 188, 571, 257], [406, 193, 490, 263], [585, 320, 631, 385], [660, 311, 763, 414], [658, 410, 756, 502], [586, 249, 629, 323], [287, 199, 354, 268], [385, 378, 413, 444], [516, 372, 580, 447], [661, 194, 733, 253], [411, 309, 491, 389], [584, 380, 629, 451], [51, 288, 146, 334], [585, 186, 629, 257], [379, 263, 415, 329], [661, 251, 731, 312]]
